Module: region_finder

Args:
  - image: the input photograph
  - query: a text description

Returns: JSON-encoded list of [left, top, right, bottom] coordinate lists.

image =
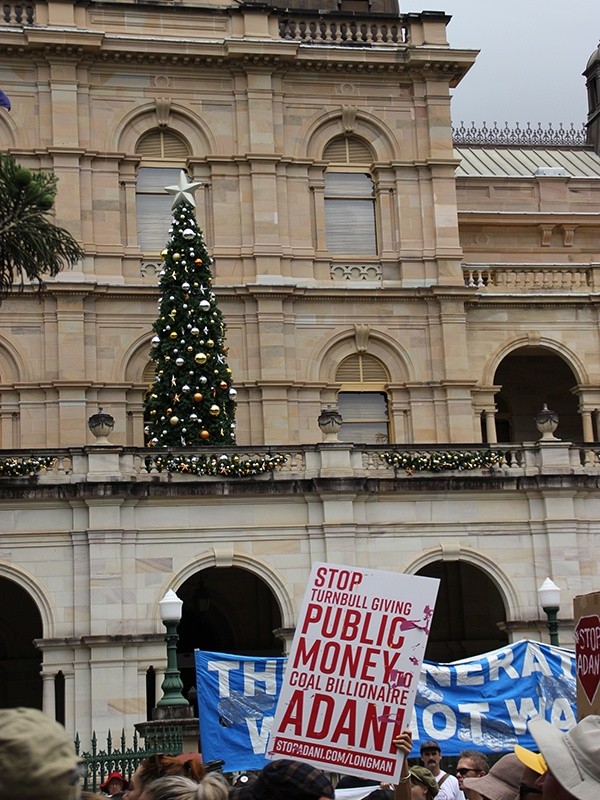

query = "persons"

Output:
[[0, 708, 600, 800]]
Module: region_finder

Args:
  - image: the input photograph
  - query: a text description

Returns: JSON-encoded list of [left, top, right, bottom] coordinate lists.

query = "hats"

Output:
[[420, 740, 441, 754], [409, 766, 439, 792], [463, 753, 527, 800], [527, 714, 600, 800], [99, 772, 127, 791], [515, 744, 548, 775], [228, 772, 259, 791], [0, 707, 86, 783], [261, 758, 336, 800]]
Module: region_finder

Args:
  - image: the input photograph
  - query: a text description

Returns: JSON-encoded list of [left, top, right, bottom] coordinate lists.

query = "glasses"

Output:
[[148, 750, 166, 777], [457, 767, 482, 777]]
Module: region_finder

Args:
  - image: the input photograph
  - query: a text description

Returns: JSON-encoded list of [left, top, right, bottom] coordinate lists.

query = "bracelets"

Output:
[[399, 771, 411, 784]]
